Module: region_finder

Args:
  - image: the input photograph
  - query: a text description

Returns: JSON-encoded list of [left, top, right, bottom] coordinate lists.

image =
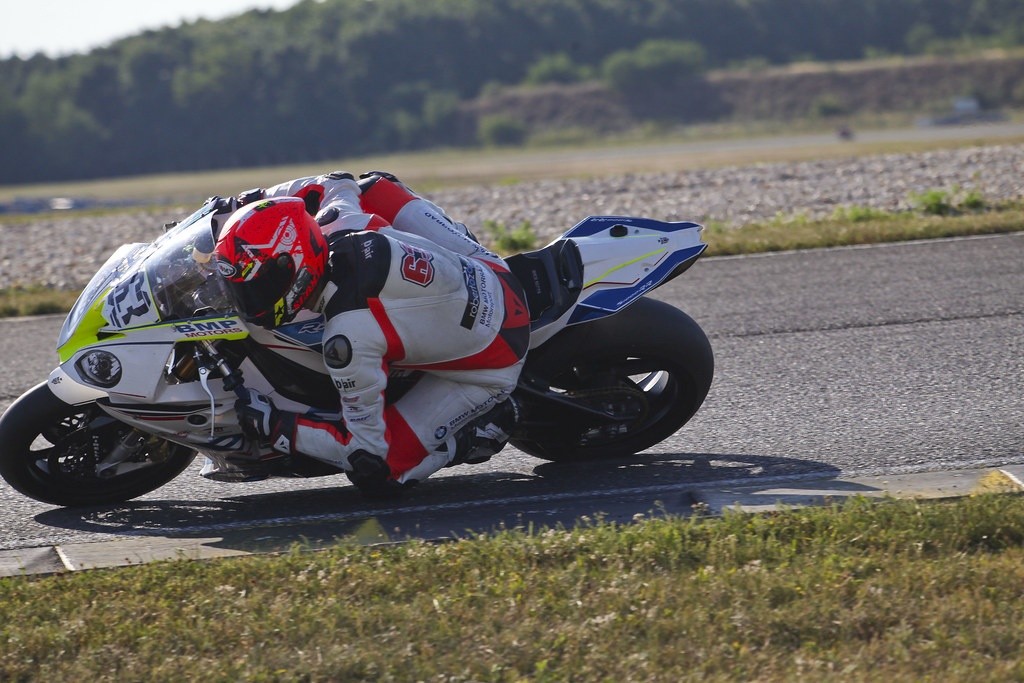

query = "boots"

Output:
[[441, 395, 519, 467]]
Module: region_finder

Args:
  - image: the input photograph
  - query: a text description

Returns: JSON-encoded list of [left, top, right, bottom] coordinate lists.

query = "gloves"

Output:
[[233, 388, 297, 460], [203, 188, 263, 241]]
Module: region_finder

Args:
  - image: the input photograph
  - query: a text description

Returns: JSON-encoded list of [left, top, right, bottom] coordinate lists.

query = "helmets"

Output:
[[215, 196, 329, 331]]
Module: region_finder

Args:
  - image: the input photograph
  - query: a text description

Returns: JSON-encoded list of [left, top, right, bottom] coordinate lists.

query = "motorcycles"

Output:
[[0, 195, 717, 512]]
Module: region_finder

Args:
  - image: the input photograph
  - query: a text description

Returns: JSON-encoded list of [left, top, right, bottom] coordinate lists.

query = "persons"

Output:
[[212, 170, 531, 500]]
